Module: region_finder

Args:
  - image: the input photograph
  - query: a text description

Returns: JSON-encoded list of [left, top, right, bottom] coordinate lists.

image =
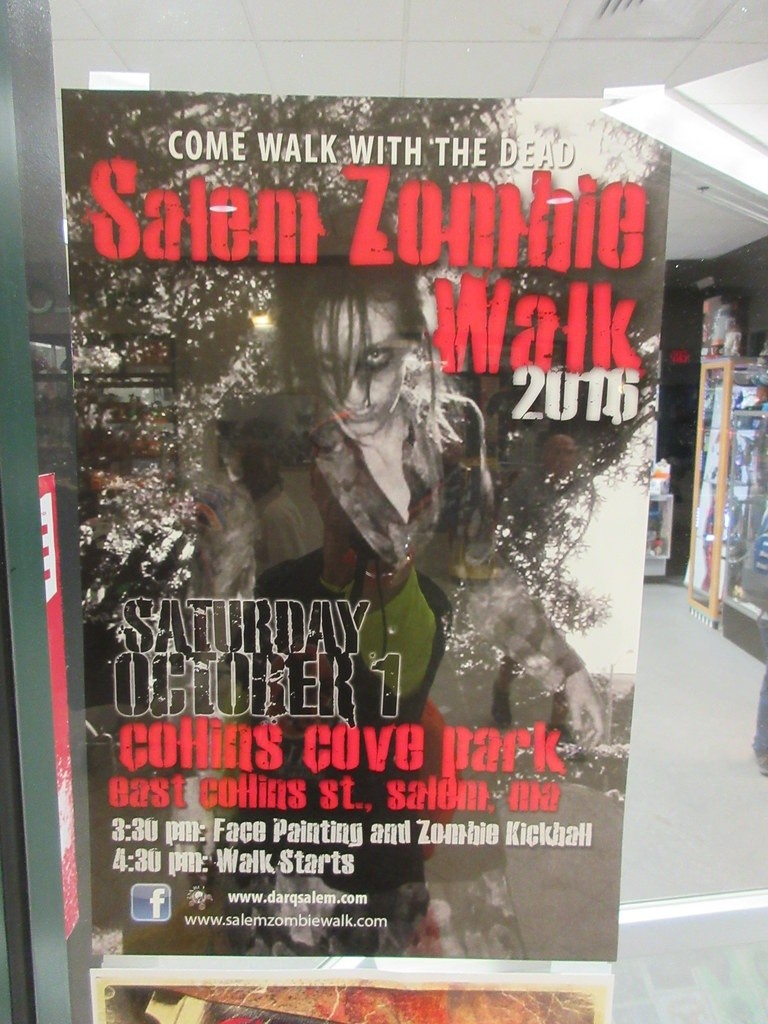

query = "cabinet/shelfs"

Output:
[[686, 355, 768, 631], [644, 493, 674, 584]]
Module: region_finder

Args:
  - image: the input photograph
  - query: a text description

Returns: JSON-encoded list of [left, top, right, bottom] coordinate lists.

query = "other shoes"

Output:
[[754, 750, 768, 775]]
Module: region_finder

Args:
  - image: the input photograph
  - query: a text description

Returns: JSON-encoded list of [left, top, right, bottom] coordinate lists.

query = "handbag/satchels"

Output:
[[753, 511, 768, 575]]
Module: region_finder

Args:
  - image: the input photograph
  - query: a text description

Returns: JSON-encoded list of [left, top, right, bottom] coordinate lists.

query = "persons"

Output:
[[188, 206, 605, 957]]
